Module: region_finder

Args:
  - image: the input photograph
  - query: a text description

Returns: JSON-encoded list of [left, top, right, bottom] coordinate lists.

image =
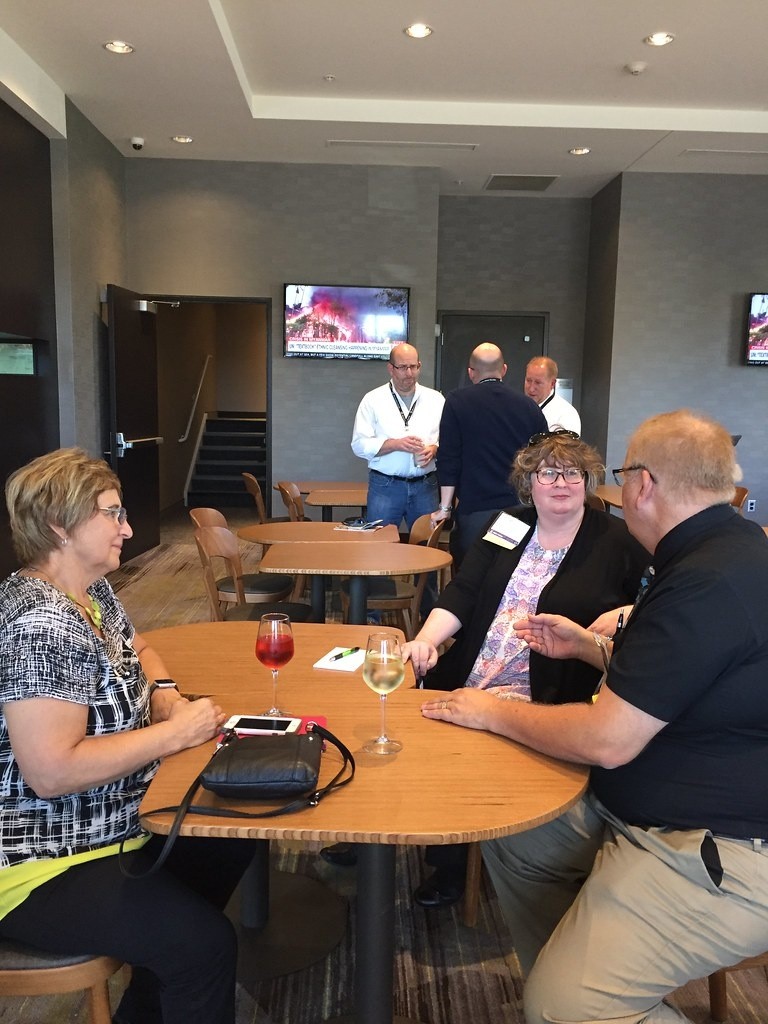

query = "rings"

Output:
[[428, 458, 432, 462], [441, 701, 447, 709]]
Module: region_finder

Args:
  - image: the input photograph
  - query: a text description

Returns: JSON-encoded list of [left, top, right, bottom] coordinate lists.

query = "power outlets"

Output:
[[748, 500, 756, 512]]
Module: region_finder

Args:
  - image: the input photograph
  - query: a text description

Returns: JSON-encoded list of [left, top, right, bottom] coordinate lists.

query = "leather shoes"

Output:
[[317, 840, 358, 868], [412, 867, 466, 909]]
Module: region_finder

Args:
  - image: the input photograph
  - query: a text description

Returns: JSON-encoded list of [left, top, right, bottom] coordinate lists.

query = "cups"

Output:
[[413, 444, 427, 468]]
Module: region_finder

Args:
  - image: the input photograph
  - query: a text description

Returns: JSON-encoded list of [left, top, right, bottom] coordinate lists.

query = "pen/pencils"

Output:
[[490, 529, 519, 546], [330, 647, 360, 662], [616, 609, 624, 636]]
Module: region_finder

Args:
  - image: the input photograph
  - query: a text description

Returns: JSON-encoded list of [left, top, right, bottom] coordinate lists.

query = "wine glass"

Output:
[[363, 632, 405, 755], [257, 613, 295, 718]]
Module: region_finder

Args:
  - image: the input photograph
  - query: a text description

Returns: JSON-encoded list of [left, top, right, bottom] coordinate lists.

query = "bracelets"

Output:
[[438, 502, 452, 512]]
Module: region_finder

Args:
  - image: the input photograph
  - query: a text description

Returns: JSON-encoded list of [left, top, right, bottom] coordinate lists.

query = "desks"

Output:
[[258, 542, 452, 625], [593, 485, 624, 509], [138, 622, 415, 980], [237, 522, 399, 624], [137, 690, 589, 1024], [273, 481, 369, 523], [304, 490, 368, 524]]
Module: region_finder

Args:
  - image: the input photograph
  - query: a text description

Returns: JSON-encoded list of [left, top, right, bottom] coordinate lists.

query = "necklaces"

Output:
[[27, 566, 102, 629]]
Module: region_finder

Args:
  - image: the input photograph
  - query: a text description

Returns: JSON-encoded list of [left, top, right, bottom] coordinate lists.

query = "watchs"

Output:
[[150, 678, 179, 696]]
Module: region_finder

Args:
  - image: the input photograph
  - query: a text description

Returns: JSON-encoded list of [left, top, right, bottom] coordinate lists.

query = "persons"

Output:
[[420, 408, 768, 1024], [524, 356, 580, 442], [0, 446, 239, 1024], [350, 342, 446, 627], [319, 424, 647, 907], [430, 342, 549, 581]]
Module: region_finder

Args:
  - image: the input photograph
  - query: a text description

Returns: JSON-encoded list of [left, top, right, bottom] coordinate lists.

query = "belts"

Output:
[[370, 469, 436, 482]]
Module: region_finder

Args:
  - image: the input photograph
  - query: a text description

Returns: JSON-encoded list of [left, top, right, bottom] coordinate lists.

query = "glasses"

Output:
[[98, 507, 128, 525], [532, 466, 587, 485], [527, 430, 581, 448], [389, 362, 419, 372], [612, 466, 657, 487]]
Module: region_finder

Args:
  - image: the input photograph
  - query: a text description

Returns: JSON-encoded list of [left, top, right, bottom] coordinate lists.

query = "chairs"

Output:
[[243, 472, 287, 574], [0, 948, 131, 1024], [190, 507, 293, 611], [195, 527, 313, 623], [730, 486, 749, 516], [339, 514, 446, 641], [278, 481, 350, 611]]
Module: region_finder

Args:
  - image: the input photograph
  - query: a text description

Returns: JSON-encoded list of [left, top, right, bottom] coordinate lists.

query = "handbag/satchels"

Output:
[[199, 732, 323, 797]]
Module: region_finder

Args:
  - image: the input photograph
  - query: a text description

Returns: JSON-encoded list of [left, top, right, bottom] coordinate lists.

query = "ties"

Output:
[[620, 564, 655, 639]]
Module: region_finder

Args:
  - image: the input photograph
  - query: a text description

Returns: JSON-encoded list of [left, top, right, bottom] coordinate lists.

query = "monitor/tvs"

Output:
[[744, 292, 768, 366], [283, 283, 410, 361]]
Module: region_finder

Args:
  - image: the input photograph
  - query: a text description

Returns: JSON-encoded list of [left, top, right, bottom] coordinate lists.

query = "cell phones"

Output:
[[224, 715, 302, 735]]
